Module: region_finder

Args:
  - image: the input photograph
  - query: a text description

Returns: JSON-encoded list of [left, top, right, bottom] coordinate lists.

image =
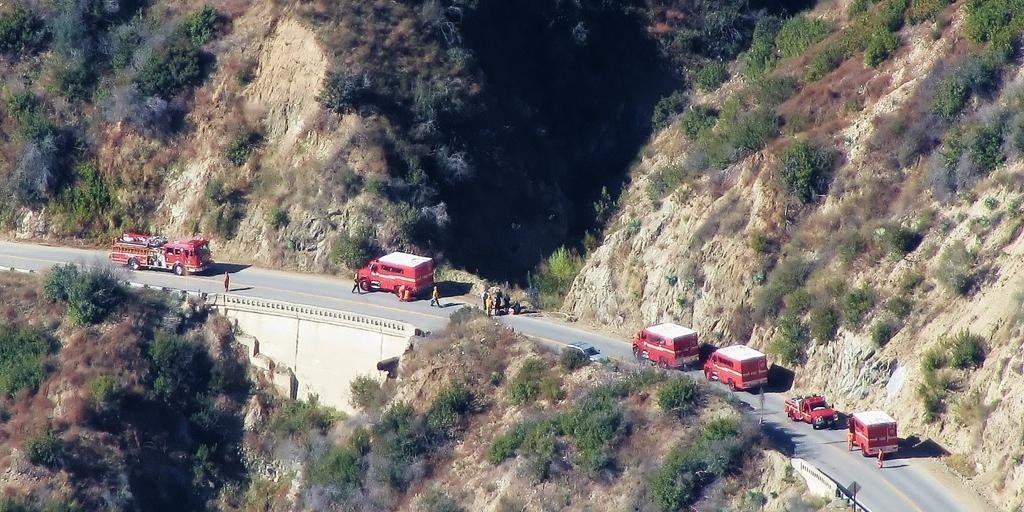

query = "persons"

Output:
[[877, 448, 884, 469], [482, 289, 521, 317], [398, 285, 405, 302], [848, 434, 852, 451], [429, 286, 440, 307], [224, 271, 229, 292], [403, 289, 411, 302], [351, 273, 360, 294]]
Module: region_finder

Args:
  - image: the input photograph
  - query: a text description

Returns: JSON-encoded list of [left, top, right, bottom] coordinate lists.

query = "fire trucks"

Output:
[[629, 322, 701, 371], [849, 408, 899, 458], [783, 394, 839, 428], [703, 344, 769, 392], [354, 251, 436, 298], [108, 232, 215, 275]]
